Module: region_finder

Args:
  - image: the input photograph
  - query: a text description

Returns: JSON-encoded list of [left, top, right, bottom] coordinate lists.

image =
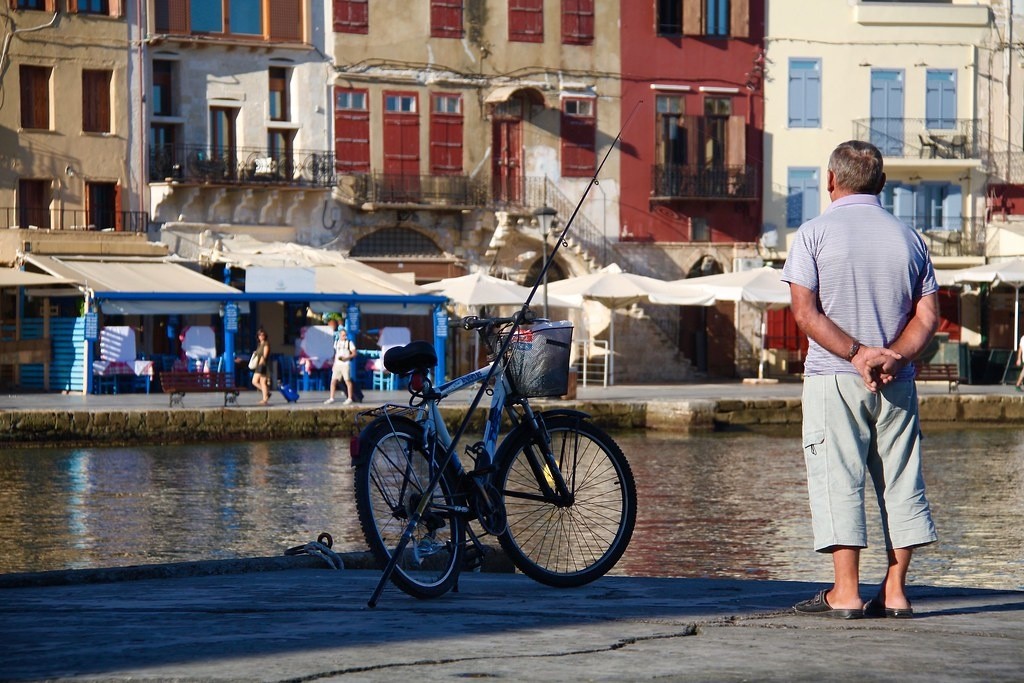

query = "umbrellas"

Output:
[[952, 256, 1024, 352], [671, 265, 791, 379], [425, 263, 716, 388]]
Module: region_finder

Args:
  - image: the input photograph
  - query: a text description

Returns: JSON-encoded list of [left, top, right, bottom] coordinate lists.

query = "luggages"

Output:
[[276, 384, 300, 403], [339, 377, 364, 403]]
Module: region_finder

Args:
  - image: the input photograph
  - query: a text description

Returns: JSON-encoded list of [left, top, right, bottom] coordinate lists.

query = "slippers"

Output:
[[864, 590, 913, 619], [792, 589, 863, 618]]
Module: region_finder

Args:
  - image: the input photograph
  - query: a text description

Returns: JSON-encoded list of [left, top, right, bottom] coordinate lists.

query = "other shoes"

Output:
[[258, 393, 272, 404], [343, 399, 352, 405], [324, 399, 333, 404]]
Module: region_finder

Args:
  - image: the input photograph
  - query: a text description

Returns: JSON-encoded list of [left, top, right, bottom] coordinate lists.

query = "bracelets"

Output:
[[844, 337, 861, 362]]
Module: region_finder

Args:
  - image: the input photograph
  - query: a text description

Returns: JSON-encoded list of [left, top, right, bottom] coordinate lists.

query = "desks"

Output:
[[170, 359, 187, 371], [295, 355, 334, 392], [365, 358, 385, 389], [92, 360, 155, 395]]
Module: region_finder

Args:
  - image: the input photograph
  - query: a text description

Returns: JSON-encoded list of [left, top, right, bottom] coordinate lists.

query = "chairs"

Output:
[[942, 231, 964, 257], [91, 351, 417, 396], [918, 134, 967, 159]]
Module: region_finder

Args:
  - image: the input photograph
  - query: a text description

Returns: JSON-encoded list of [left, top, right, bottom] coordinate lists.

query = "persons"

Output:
[[1015, 335, 1024, 393], [325, 330, 357, 405], [781, 140, 941, 619], [251, 329, 272, 406]]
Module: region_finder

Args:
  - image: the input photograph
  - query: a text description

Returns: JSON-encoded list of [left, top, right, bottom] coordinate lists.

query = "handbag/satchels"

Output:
[[248, 351, 259, 369]]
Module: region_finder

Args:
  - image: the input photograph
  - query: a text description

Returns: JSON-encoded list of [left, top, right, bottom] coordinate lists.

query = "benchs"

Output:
[[913, 362, 967, 394], [159, 372, 246, 408]]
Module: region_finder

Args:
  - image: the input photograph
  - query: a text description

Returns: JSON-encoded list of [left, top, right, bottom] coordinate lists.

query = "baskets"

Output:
[[487, 326, 574, 398]]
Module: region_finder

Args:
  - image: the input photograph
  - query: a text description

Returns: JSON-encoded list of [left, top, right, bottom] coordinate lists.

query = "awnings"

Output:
[[0, 267, 78, 285], [214, 258, 431, 316], [29, 256, 249, 315]]
